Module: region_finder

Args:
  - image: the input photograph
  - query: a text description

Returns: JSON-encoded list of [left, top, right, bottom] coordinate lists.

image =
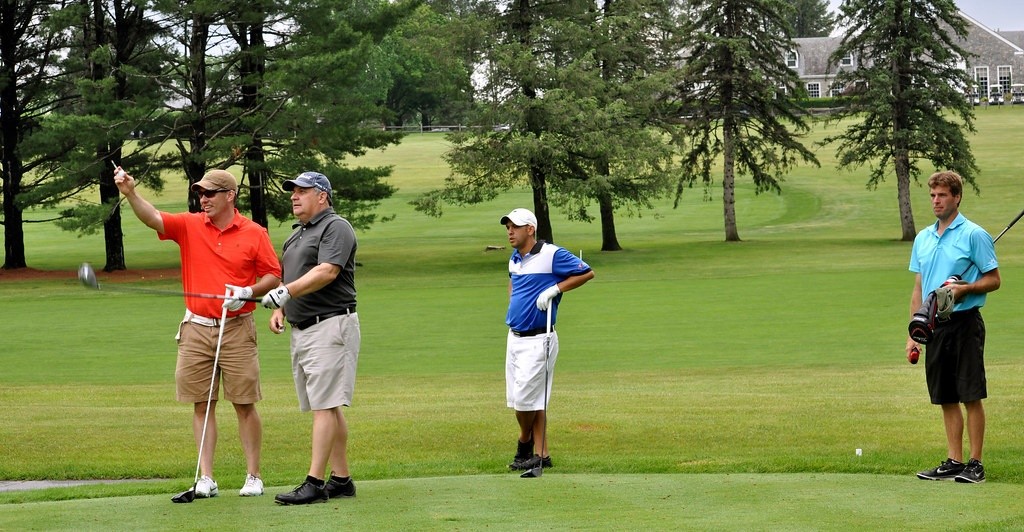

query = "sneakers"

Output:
[[188, 474, 219, 497], [323, 472, 356, 498], [917, 459, 966, 480], [274, 481, 330, 506], [508, 433, 535, 471], [239, 474, 264, 497], [517, 453, 554, 470], [955, 459, 986, 484]]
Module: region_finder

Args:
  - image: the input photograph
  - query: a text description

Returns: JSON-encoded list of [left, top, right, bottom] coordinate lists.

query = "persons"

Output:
[[501, 208, 596, 470], [905, 171, 1002, 484], [262, 171, 360, 505], [115, 165, 280, 497]]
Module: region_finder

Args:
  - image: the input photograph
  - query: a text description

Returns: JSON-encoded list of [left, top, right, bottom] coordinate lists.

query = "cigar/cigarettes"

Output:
[[111, 160, 118, 169]]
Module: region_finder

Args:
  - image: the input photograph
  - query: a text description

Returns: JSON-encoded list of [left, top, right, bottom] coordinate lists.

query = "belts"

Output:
[[511, 325, 554, 337], [290, 307, 357, 330]]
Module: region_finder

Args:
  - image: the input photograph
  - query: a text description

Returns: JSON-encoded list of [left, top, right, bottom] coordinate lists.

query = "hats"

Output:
[[282, 171, 332, 198], [500, 207, 537, 232], [192, 169, 237, 195]]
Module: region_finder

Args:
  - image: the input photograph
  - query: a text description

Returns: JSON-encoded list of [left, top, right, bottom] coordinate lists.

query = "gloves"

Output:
[[261, 286, 292, 309], [537, 283, 561, 311], [222, 283, 253, 312]]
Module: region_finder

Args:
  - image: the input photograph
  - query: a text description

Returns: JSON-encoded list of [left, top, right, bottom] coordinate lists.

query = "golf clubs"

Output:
[[76, 259, 284, 310], [521, 297, 553, 481], [958, 211, 1024, 278], [170, 283, 233, 504]]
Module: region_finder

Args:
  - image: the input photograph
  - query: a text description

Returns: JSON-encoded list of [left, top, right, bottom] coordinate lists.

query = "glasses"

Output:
[[198, 189, 231, 198]]
